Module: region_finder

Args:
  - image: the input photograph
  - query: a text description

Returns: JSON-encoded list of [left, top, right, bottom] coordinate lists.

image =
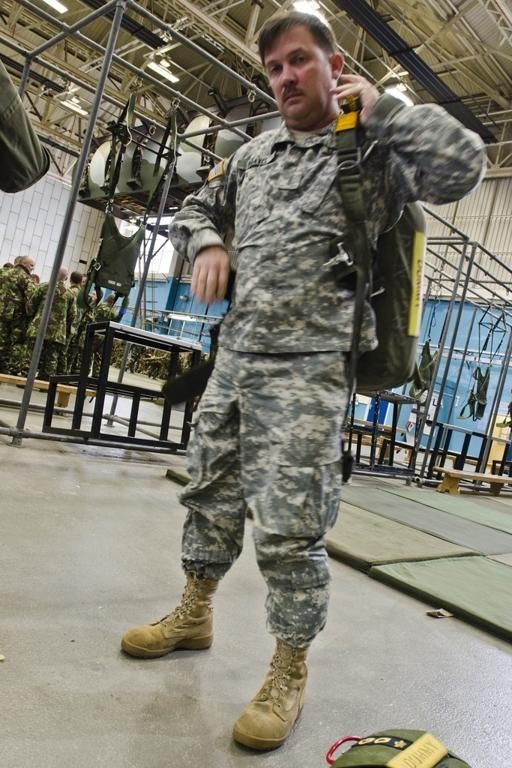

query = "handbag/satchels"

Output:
[[326, 729, 466, 763]]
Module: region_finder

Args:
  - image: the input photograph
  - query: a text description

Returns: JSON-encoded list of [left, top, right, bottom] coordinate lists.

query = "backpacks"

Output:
[[335, 111, 426, 389]]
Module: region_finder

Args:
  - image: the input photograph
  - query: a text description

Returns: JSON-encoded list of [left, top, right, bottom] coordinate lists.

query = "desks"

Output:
[[72, 321, 202, 450], [426, 420, 511, 489], [345, 389, 420, 468]]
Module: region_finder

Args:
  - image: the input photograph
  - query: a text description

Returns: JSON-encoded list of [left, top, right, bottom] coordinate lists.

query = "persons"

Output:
[[120, 10, 485, 753], [108, 320, 209, 382], [0, 255, 120, 392], [0, 54, 49, 194]]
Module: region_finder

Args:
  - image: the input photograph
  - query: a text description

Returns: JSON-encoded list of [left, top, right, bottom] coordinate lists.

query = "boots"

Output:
[[121, 573, 214, 658], [233, 639, 308, 750]]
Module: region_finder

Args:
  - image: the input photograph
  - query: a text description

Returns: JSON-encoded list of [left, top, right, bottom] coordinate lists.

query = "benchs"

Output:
[[379, 438, 512, 497], [342, 416, 407, 467], [0, 375, 96, 407], [41, 375, 176, 444]]
